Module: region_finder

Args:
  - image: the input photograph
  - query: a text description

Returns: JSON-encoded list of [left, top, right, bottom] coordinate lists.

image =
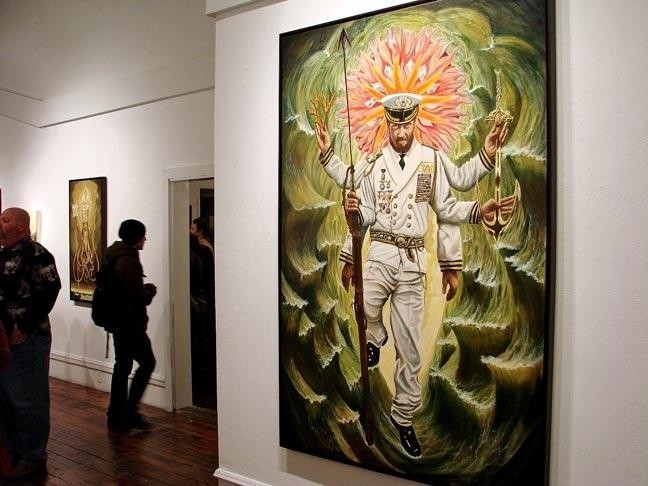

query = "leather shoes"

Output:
[[389, 415, 422, 458], [367, 334, 388, 369], [8, 461, 45, 475]]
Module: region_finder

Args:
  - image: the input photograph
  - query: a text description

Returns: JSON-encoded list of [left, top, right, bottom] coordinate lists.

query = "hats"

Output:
[[381, 93, 422, 124]]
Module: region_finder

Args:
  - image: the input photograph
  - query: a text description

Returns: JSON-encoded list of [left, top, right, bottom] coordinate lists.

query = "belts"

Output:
[[369, 230, 426, 261]]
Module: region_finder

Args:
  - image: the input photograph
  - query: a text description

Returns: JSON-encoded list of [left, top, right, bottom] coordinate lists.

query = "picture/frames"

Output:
[[277, 0, 556, 486], [69, 177, 108, 302]]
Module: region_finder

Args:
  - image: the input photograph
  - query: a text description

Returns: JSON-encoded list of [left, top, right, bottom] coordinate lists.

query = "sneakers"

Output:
[[109, 411, 155, 433]]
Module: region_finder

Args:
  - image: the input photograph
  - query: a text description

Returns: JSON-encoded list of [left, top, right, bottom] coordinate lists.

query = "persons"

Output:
[[0, 206, 62, 480], [101, 219, 157, 436], [189, 215, 216, 391]]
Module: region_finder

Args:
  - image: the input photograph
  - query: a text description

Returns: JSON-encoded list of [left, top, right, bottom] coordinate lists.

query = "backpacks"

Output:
[[93, 252, 124, 327]]
